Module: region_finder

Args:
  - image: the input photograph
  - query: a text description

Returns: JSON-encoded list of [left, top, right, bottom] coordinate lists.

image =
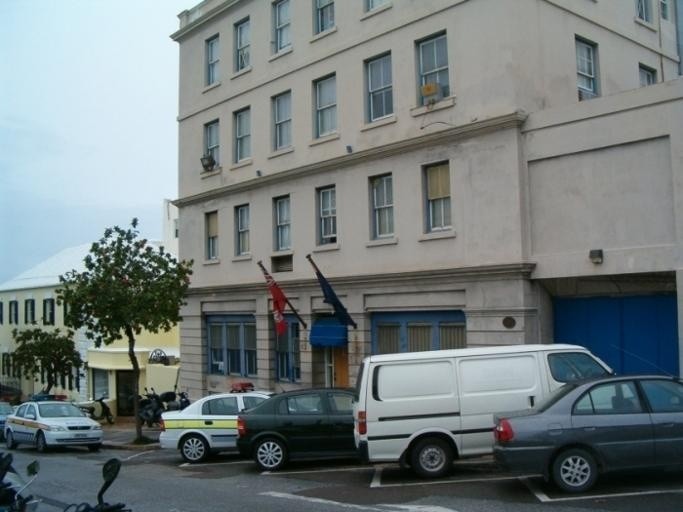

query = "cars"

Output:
[[236, 385, 357, 472], [492, 374, 681, 493], [158, 383, 317, 462], [4, 393, 104, 453], [0, 402, 15, 442]]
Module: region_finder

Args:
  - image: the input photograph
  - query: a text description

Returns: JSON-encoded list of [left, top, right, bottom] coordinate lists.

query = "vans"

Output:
[[351, 341, 619, 479]]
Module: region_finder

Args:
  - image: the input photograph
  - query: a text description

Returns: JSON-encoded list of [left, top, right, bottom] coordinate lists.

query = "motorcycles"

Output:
[[77, 391, 116, 424], [128, 387, 190, 428]]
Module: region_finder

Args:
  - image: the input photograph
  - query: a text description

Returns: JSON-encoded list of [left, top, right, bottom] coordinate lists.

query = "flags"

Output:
[[315, 272, 354, 325], [261, 267, 287, 334]]
